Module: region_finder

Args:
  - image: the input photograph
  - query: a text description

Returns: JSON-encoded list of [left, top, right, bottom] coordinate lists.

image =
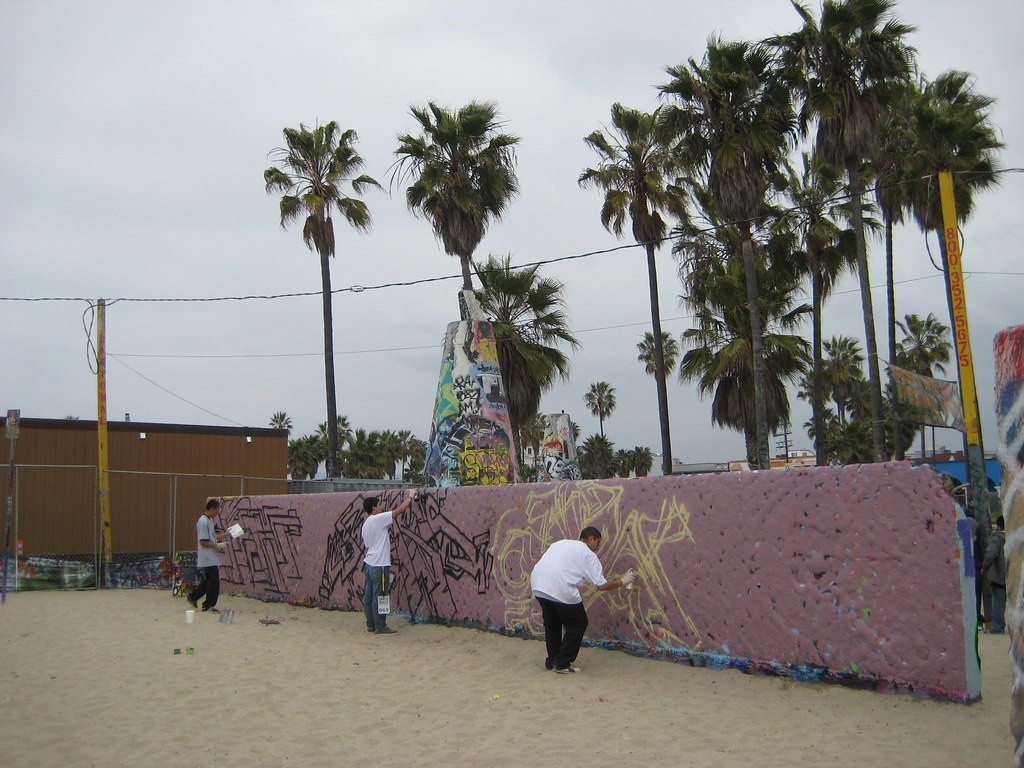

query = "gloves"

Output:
[[409, 488, 418, 499], [620, 567, 635, 586], [216, 542, 229, 551]]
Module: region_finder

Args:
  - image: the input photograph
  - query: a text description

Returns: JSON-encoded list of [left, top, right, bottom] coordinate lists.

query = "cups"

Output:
[[186, 610, 195, 624]]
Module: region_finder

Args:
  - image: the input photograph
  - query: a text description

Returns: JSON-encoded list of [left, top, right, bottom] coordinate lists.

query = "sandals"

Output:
[[554, 666, 580, 673]]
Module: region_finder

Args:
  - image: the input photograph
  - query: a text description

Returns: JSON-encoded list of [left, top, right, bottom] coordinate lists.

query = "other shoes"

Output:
[[202, 607, 219, 614], [184, 595, 198, 610]]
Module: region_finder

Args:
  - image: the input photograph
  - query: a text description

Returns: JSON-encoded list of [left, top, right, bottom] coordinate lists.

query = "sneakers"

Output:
[[366, 626, 375, 633], [374, 624, 398, 635]]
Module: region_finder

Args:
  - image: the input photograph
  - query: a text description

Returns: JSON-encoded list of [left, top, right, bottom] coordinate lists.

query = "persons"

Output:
[[361, 488, 419, 636], [530, 526, 636, 673], [962, 485, 1007, 635], [187, 499, 232, 614]]
